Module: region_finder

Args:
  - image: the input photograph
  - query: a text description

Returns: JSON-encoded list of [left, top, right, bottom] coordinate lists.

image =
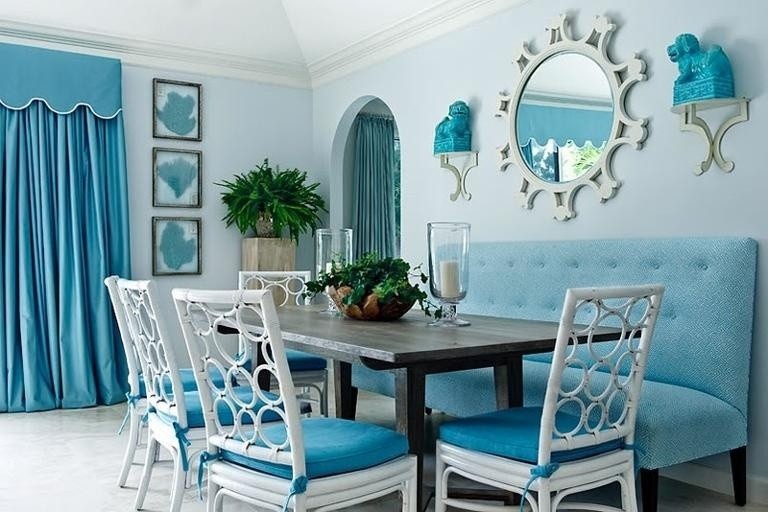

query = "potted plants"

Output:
[[301, 251, 451, 321], [213, 159, 329, 246]]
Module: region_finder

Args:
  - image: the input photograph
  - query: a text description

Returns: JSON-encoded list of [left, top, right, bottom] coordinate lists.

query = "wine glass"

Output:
[[316, 228, 353, 312], [426, 222, 472, 327]]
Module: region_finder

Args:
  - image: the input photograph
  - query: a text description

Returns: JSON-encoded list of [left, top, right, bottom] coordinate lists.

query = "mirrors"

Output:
[[492, 12, 647, 222]]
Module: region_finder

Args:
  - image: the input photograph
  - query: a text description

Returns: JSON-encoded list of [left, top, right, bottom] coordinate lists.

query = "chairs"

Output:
[[173, 290, 417, 512], [106, 275, 235, 490], [118, 279, 311, 511], [237, 270, 329, 418], [435, 284, 666, 512]]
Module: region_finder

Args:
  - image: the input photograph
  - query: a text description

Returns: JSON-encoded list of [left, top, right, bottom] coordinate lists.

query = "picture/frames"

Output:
[[151, 78, 202, 140], [152, 216, 202, 275], [152, 147, 203, 209]]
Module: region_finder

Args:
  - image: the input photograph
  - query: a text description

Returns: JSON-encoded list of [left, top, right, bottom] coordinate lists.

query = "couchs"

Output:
[[351, 237, 757, 512]]
